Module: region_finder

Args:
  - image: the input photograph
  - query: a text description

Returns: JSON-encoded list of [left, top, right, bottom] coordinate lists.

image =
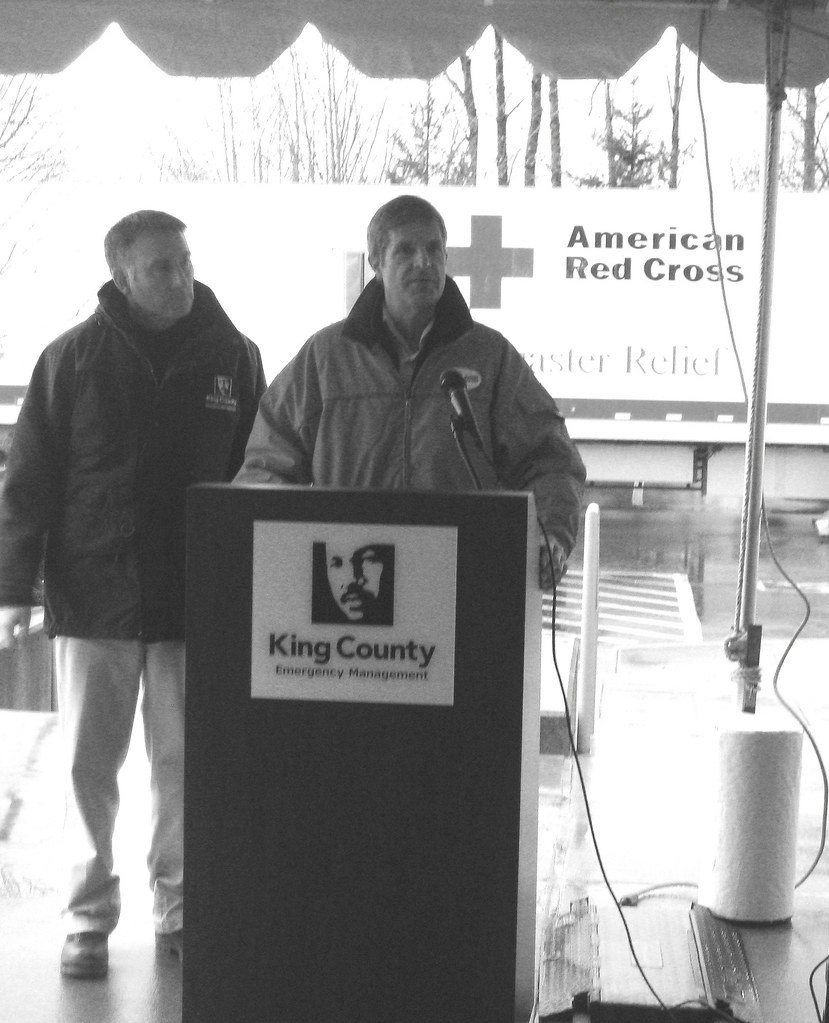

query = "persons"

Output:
[[0, 212, 267, 978], [237, 194, 586, 592], [323, 544, 392, 625]]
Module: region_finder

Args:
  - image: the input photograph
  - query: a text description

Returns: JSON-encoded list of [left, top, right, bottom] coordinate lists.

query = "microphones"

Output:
[[440, 369, 483, 450]]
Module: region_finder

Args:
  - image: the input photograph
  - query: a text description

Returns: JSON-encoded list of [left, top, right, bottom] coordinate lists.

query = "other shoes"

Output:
[[156, 929, 185, 962], [61, 933, 107, 978]]
